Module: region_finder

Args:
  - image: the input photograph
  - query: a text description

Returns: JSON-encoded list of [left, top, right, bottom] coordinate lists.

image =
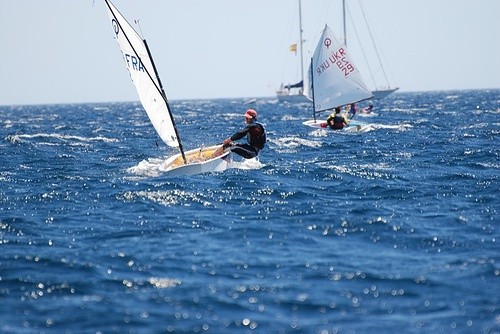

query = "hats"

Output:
[[244, 109, 257, 119]]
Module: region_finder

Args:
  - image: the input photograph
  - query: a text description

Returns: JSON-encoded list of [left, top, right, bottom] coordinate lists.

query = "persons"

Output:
[[346, 101, 358, 123], [321, 106, 349, 132], [209, 110, 265, 161], [366, 101, 374, 111]]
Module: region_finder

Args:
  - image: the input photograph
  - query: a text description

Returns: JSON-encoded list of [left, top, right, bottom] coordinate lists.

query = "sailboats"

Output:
[[375, 134, 375, 137], [104, 0, 232, 178], [276, 1, 400, 102], [303, 22, 377, 136]]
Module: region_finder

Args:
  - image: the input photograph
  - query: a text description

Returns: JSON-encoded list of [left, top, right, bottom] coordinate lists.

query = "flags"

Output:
[[289, 43, 297, 52]]
